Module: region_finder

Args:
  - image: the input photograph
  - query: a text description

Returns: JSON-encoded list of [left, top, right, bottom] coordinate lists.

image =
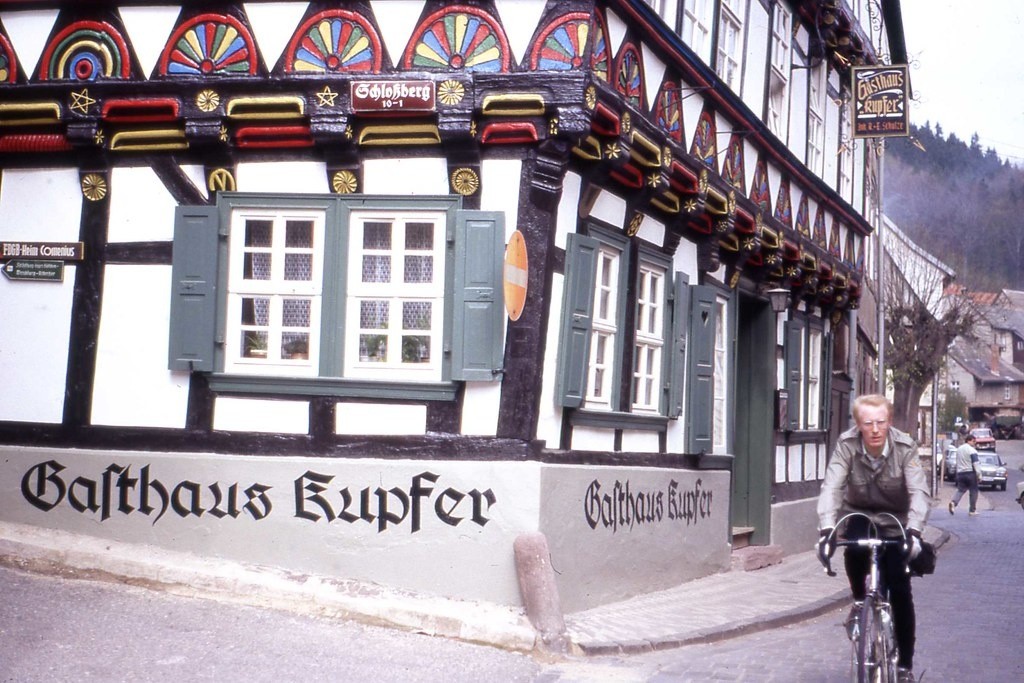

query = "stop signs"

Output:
[[504, 227, 533, 326]]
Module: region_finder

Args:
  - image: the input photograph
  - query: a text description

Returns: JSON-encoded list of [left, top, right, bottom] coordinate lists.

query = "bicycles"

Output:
[[815, 511, 921, 683]]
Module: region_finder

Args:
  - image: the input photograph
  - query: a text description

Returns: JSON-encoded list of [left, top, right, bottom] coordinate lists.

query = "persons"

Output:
[[949, 435, 983, 516], [816, 394, 931, 683], [958, 426, 966, 441]]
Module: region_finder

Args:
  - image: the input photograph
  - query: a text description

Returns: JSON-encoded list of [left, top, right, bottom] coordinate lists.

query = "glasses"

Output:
[[863, 419, 889, 432]]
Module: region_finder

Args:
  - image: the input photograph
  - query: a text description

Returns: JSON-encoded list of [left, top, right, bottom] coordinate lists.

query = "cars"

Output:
[[937, 438, 960, 481], [972, 451, 1008, 491], [968, 427, 996, 452]]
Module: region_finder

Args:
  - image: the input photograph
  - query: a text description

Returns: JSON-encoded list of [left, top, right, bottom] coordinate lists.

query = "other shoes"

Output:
[[845, 603, 866, 641], [948, 501, 955, 515], [898, 668, 915, 683], [969, 510, 979, 516]]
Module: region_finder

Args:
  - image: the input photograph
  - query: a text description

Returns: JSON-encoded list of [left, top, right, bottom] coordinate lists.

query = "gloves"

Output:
[[902, 528, 923, 565], [815, 527, 837, 568]]
